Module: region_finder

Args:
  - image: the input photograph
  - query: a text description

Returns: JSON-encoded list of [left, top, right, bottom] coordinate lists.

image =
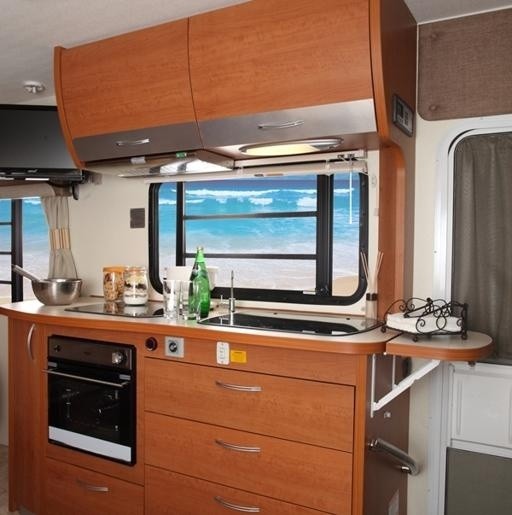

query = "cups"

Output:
[[180, 279, 201, 319], [162, 280, 179, 318]]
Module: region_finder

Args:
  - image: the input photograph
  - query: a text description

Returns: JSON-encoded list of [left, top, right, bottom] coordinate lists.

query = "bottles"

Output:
[[364, 293, 378, 319], [190, 245, 210, 316], [102, 301, 151, 316], [102, 265, 148, 306]]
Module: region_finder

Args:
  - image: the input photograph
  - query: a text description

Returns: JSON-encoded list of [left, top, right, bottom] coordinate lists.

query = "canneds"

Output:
[[123, 267, 148, 305], [102, 266, 124, 302], [103, 302, 124, 315], [123, 305, 148, 318]]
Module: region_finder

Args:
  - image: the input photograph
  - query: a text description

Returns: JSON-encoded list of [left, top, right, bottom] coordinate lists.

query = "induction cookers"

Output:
[[66, 299, 164, 318]]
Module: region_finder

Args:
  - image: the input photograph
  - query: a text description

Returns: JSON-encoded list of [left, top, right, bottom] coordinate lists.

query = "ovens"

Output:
[[46, 334, 136, 467]]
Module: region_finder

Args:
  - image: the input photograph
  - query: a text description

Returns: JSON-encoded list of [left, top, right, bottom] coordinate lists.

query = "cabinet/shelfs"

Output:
[[189, 1, 419, 147], [143, 352, 413, 515], [54, 17, 203, 168], [7, 319, 44, 515], [43, 458, 144, 515]]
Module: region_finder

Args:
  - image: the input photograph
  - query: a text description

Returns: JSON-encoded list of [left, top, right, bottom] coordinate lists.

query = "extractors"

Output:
[[78, 150, 235, 179]]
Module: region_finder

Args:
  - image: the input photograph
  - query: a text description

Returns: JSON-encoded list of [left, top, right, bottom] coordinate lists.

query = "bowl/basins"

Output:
[[31, 277, 83, 305], [165, 267, 219, 308]]
